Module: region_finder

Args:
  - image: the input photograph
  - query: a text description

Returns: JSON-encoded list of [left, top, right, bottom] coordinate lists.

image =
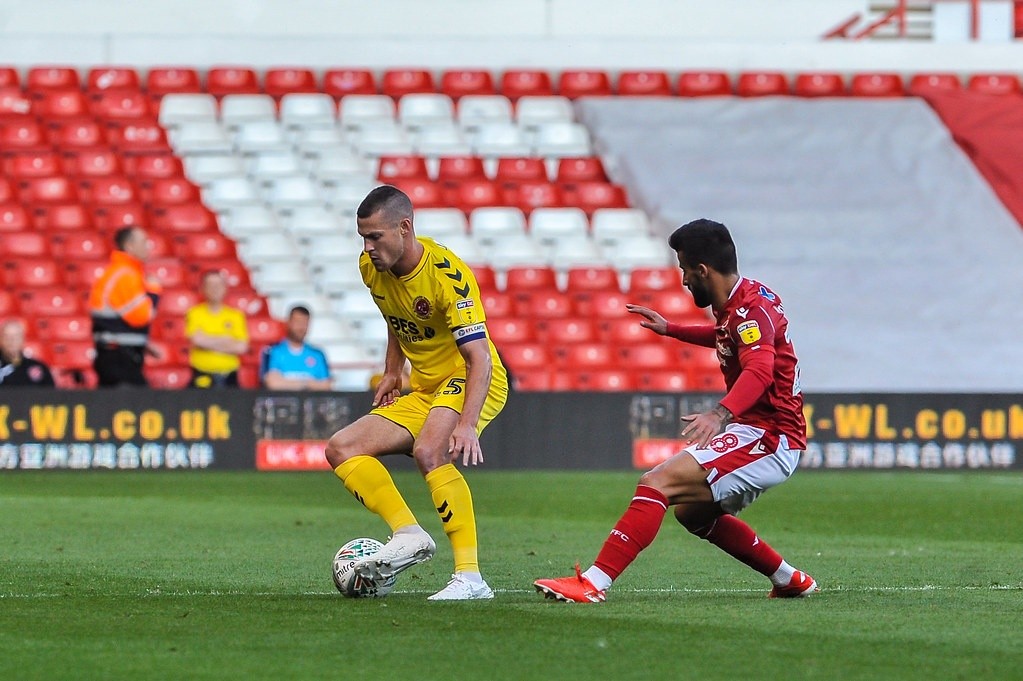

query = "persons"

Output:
[[323, 185, 509, 601], [259, 307, 333, 391], [182, 268, 249, 391], [0, 314, 54, 388], [83, 224, 161, 390], [533, 218, 817, 604]]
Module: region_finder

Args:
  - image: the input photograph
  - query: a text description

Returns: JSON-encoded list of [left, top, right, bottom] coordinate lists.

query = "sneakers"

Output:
[[354, 525, 436, 581], [533, 563, 606, 604], [767, 570, 818, 598], [426, 573, 494, 600]]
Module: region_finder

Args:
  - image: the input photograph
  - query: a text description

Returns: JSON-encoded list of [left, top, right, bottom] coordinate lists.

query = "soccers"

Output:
[[332, 538, 396, 599]]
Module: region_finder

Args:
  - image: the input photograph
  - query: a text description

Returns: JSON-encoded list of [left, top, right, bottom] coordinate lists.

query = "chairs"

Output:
[[0, 66, 730, 390], [737, 73, 1023, 226]]
